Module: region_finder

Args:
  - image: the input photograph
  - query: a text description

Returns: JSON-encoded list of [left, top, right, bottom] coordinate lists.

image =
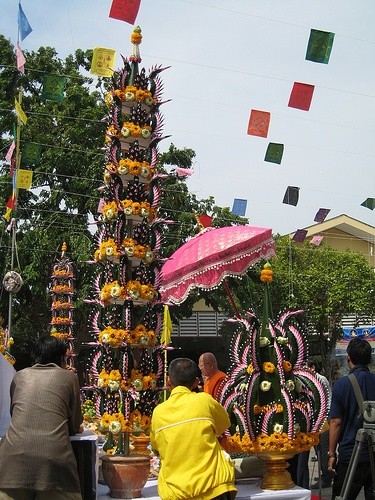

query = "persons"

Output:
[[289, 450, 310, 490], [0, 335, 84, 500], [150, 357, 238, 500], [327, 341, 375, 500], [308, 355, 337, 489], [199, 352, 227, 400]]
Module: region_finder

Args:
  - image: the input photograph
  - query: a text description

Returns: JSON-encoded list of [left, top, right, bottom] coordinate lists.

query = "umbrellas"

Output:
[[157, 214, 277, 320]]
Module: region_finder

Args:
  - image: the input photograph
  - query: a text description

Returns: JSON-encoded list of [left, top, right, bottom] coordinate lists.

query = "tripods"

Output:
[[334, 401, 375, 500]]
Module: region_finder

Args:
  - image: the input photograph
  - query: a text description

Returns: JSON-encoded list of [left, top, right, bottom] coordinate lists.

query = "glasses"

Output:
[[198, 363, 209, 368]]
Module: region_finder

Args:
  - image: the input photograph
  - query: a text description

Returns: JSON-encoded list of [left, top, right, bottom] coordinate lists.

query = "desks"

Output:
[[97, 480, 311, 500]]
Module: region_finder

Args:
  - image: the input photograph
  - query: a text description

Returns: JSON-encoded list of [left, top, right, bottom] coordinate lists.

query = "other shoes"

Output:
[[311, 457, 318, 462], [311, 481, 332, 489], [314, 476, 319, 481]]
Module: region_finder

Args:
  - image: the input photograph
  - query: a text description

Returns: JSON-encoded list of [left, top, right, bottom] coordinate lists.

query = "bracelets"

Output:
[[328, 452, 336, 457]]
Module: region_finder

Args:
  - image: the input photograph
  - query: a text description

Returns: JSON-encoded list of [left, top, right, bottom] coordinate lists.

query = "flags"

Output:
[[199, 199, 247, 228], [89, 48, 116, 77], [110, 0, 142, 24], [177, 167, 194, 176], [247, 29, 335, 221], [3, 4, 33, 235], [292, 229, 324, 246], [361, 198, 375, 211]]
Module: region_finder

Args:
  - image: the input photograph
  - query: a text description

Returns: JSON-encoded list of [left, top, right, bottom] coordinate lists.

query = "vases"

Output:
[[257, 452, 298, 490], [99, 454, 153, 499]]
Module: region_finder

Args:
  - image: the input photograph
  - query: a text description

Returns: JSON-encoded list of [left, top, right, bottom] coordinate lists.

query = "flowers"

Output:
[[129, 55, 142, 63], [97, 368, 157, 392], [104, 158, 155, 182], [49, 241, 77, 358], [104, 86, 158, 108], [147, 445, 160, 472], [95, 237, 154, 263], [99, 280, 158, 303], [221, 269, 320, 455], [102, 199, 155, 223], [104, 122, 152, 143], [131, 31, 142, 44], [98, 324, 155, 348], [94, 409, 151, 435]]
